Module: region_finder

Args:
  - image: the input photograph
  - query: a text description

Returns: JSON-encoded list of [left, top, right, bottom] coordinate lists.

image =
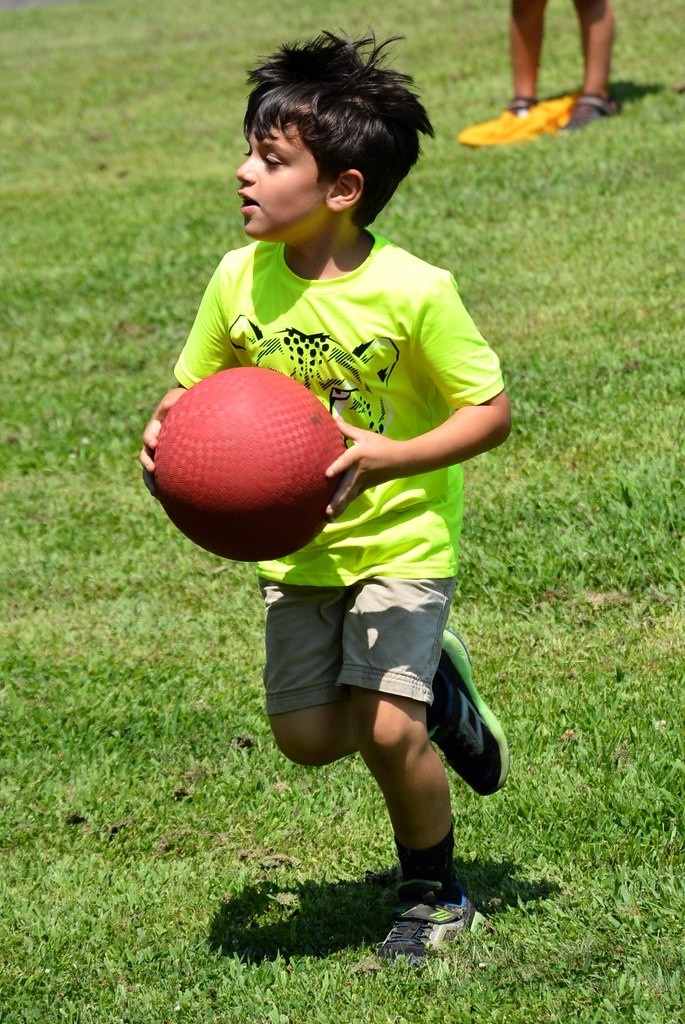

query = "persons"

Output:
[[501, 0, 619, 133], [136, 31, 516, 979]]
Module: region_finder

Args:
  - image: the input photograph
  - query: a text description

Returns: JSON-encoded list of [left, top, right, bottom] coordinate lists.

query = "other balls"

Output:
[[151, 364, 346, 563]]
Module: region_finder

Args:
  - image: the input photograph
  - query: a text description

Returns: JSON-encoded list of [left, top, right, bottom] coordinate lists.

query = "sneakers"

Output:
[[424, 628, 510, 795], [375, 890, 487, 961]]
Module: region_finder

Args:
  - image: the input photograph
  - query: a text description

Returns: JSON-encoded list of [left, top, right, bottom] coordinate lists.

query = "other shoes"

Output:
[[509, 97, 537, 115], [564, 94, 618, 131]]
